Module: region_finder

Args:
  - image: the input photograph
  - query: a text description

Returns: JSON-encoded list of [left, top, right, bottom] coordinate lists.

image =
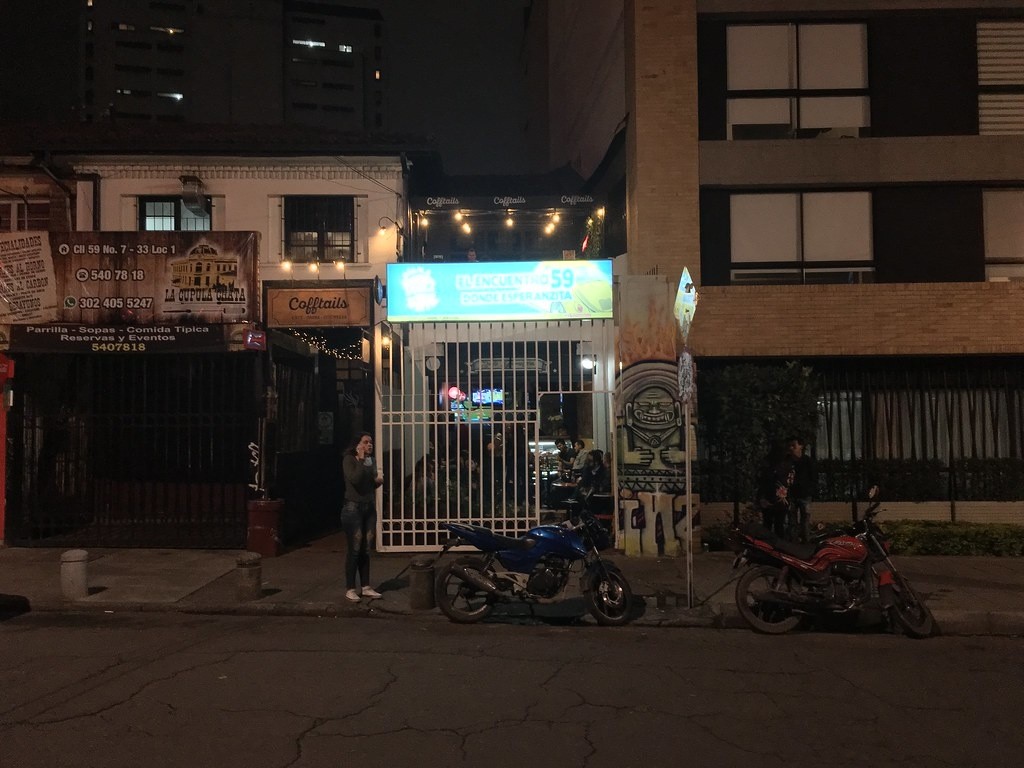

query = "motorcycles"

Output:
[[433, 495, 633, 626], [723, 499, 933, 639]]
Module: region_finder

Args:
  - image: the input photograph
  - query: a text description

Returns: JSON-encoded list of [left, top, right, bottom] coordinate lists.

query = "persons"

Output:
[[756, 439, 817, 541], [550, 440, 611, 506], [408, 425, 527, 491], [341, 432, 385, 601]]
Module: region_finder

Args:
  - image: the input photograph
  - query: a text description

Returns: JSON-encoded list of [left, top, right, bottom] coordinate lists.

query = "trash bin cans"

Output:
[[244, 497, 284, 558]]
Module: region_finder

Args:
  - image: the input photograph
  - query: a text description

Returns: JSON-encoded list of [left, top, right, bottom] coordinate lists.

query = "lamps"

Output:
[[282, 252, 293, 280], [378, 216, 395, 235], [309, 250, 320, 280], [336, 250, 346, 279]]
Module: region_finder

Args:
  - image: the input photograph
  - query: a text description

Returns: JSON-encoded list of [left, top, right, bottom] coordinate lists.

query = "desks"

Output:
[[553, 482, 577, 487]]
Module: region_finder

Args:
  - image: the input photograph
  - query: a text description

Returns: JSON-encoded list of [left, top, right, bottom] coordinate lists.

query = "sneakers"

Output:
[[361, 586, 382, 598], [345, 590, 360, 603]]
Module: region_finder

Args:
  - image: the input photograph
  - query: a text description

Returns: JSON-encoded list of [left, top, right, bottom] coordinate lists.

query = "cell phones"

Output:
[[356, 445, 360, 453]]
